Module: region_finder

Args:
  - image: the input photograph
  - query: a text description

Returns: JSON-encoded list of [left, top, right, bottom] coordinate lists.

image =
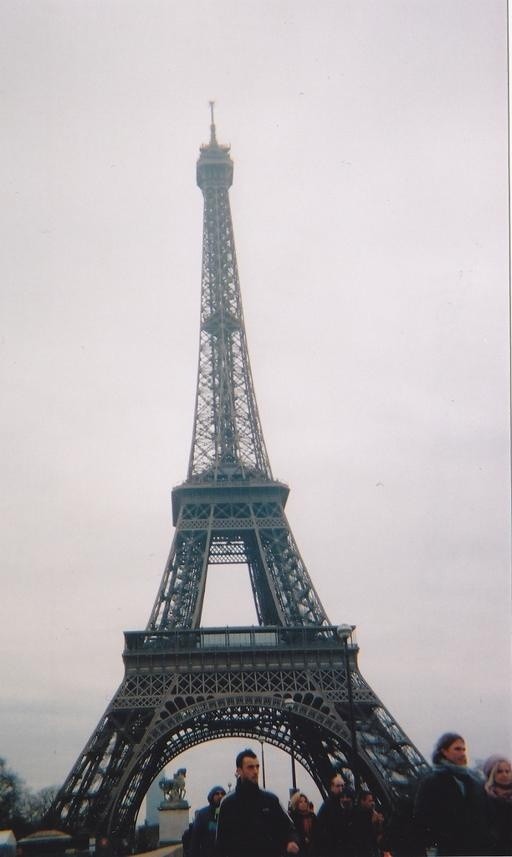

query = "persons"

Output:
[[182, 748, 385, 854], [382, 730, 511, 857]]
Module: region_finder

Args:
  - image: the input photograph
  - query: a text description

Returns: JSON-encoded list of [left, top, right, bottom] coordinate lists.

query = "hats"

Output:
[[483, 753, 512, 779]]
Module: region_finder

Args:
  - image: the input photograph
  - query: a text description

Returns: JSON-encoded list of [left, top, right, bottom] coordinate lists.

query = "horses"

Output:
[[158, 767, 188, 801]]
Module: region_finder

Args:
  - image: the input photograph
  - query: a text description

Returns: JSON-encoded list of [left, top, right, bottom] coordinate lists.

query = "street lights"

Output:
[[335, 621, 361, 796], [227, 781, 232, 793], [283, 695, 298, 794], [256, 734, 266, 790]]
